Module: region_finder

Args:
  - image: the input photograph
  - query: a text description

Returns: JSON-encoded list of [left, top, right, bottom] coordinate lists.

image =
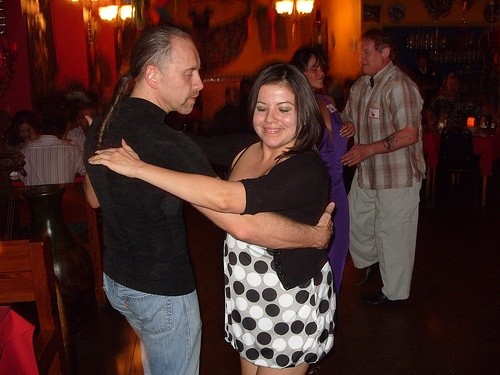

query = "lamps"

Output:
[[275, 0, 316, 22]]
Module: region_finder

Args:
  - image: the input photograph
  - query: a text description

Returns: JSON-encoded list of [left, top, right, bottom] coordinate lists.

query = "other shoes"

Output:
[[361, 288, 402, 305], [355, 262, 379, 284]]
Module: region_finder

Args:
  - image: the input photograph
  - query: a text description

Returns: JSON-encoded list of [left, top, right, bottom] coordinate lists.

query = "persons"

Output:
[[81, 27, 335, 375], [84, 64, 342, 375], [338, 29, 426, 309], [288, 42, 356, 294], [413, 53, 440, 89], [0, 83, 106, 281]]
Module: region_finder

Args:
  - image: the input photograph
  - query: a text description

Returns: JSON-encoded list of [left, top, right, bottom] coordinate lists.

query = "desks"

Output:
[[420, 125, 500, 206]]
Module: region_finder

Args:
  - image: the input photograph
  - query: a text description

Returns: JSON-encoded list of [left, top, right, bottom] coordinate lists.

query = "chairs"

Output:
[[1, 234, 67, 375], [0, 181, 105, 311], [434, 123, 483, 233]]
